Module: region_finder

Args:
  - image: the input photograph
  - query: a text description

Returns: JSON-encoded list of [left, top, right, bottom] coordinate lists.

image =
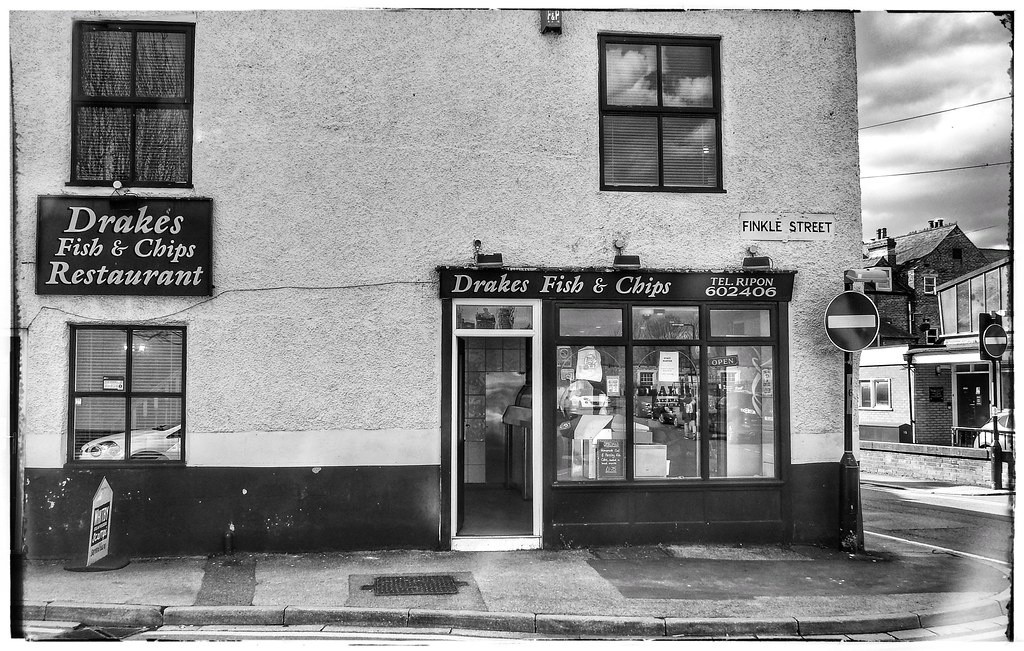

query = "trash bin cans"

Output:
[[899, 423, 913, 443]]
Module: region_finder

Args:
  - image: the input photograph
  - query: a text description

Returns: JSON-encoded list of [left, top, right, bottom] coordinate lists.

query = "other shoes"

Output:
[[684, 436, 689, 439], [693, 437, 696, 440]]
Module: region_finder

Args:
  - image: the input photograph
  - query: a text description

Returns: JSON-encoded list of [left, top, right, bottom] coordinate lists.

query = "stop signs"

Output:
[[824, 290, 881, 354], [982, 324, 1008, 358]]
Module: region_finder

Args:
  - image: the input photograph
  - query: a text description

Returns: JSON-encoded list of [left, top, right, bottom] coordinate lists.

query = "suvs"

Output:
[[973, 410, 1015, 452], [716, 380, 774, 444]]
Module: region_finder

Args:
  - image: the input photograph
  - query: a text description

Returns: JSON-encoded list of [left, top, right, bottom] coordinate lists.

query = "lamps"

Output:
[[475, 253, 504, 267], [741, 255, 770, 273], [613, 255, 640, 270]]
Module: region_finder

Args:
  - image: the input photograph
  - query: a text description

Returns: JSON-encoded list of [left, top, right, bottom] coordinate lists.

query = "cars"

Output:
[[570, 388, 608, 409], [80, 420, 181, 461], [633, 385, 654, 418]]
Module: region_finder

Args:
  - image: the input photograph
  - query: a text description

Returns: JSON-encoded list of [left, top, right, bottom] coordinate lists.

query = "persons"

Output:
[[680, 392, 698, 441]]
